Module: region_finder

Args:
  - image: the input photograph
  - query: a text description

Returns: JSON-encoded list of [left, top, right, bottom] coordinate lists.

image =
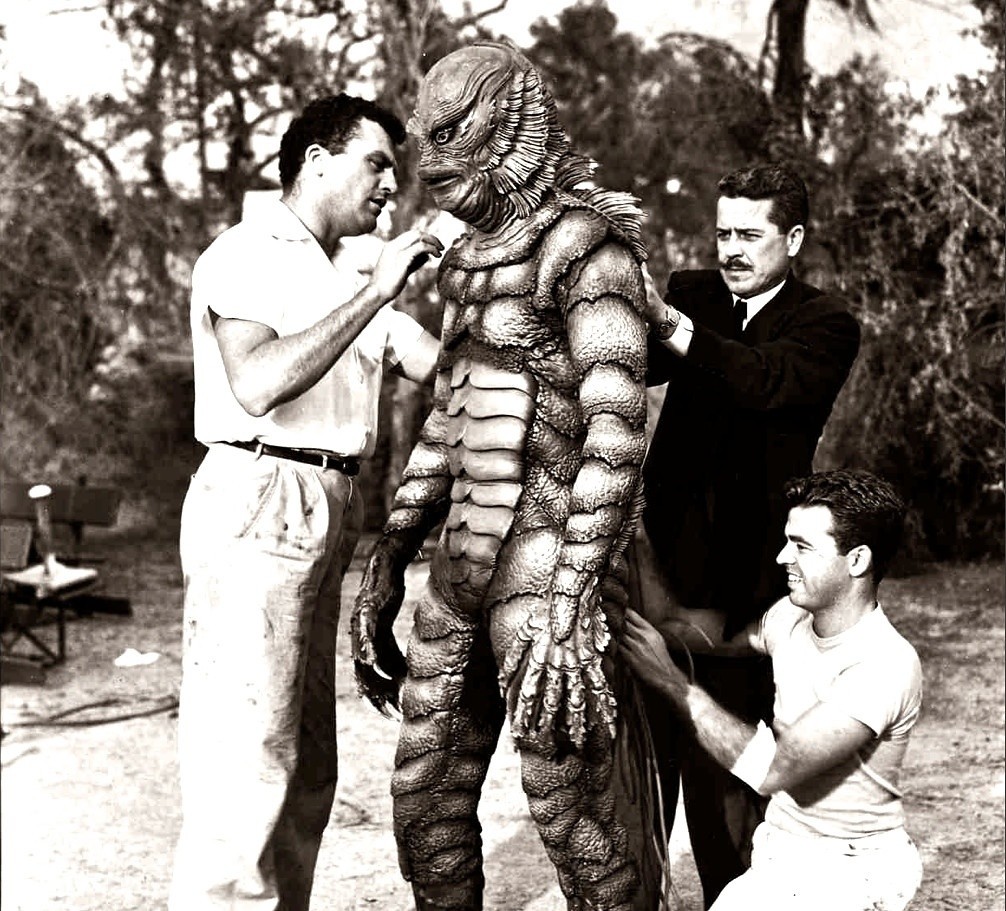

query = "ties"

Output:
[[731, 300, 748, 332]]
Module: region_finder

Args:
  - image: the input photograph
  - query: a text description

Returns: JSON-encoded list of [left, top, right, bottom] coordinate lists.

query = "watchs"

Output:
[[653, 305, 680, 340]]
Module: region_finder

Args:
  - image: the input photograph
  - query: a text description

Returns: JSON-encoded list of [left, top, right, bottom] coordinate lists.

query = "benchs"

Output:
[[0, 475, 124, 663]]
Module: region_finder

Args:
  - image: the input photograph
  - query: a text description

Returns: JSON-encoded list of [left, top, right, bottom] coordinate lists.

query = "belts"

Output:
[[216, 438, 362, 477]]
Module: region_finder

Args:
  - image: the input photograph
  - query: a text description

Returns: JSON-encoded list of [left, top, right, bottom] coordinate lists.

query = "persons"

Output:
[[642, 165, 861, 911], [165, 96, 445, 911], [616, 469, 922, 911]]
